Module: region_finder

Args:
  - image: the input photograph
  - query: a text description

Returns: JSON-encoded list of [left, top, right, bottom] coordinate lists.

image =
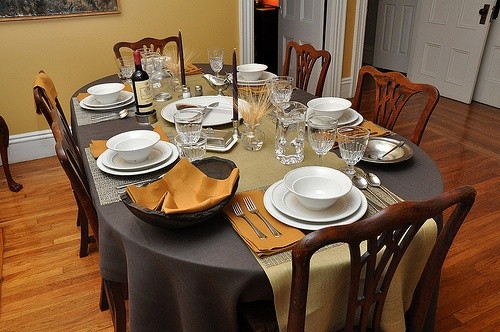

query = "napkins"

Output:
[[33, 73, 57, 115], [167, 59, 201, 74], [334, 121, 386, 143], [224, 189, 306, 258], [89, 124, 171, 158], [239, 80, 296, 95], [126, 157, 238, 214], [78, 81, 132, 102]]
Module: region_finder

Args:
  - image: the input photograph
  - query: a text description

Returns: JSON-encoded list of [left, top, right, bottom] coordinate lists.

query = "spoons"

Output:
[[353, 173, 402, 209], [91, 109, 129, 123]]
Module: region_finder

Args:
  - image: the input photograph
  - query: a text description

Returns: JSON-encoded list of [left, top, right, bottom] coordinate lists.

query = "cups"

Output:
[[276, 100, 308, 165], [173, 109, 208, 162]]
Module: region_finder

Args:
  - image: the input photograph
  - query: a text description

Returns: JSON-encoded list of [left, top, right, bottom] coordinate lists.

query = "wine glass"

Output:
[[207, 48, 224, 77], [114, 48, 166, 89], [305, 112, 370, 180], [265, 75, 295, 124]]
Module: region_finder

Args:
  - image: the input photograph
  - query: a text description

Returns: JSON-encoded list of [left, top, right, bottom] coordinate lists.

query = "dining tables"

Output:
[[70, 62, 446, 332]]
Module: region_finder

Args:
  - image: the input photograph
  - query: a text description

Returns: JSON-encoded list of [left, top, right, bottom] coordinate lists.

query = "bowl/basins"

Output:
[[307, 96, 352, 124], [283, 165, 352, 211], [105, 130, 161, 163], [237, 63, 268, 80], [120, 156, 240, 230], [355, 136, 414, 164], [86, 82, 125, 105]]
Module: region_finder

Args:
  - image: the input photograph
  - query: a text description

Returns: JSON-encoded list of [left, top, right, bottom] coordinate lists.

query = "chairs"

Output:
[[350, 66, 440, 147], [280, 39, 331, 97], [113, 35, 182, 73], [286, 184, 477, 332], [30, 71, 131, 332]]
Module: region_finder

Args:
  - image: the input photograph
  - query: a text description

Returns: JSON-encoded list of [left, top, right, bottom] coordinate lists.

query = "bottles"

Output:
[[151, 56, 175, 102], [130, 50, 154, 116], [181, 86, 203, 99]]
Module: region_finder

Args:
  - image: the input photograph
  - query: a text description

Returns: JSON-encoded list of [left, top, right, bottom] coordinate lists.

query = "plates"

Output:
[[160, 96, 251, 127], [299, 108, 364, 133], [96, 140, 179, 176], [263, 178, 368, 231], [227, 71, 278, 86], [79, 91, 135, 110]]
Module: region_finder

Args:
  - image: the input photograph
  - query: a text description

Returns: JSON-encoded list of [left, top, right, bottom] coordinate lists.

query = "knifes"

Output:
[[174, 101, 231, 123]]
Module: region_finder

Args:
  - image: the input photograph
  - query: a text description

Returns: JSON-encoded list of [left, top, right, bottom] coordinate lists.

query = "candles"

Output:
[[178, 29, 185, 84], [232, 47, 239, 120]]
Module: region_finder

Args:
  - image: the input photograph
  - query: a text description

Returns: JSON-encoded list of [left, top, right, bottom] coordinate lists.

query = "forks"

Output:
[[231, 196, 283, 239]]
